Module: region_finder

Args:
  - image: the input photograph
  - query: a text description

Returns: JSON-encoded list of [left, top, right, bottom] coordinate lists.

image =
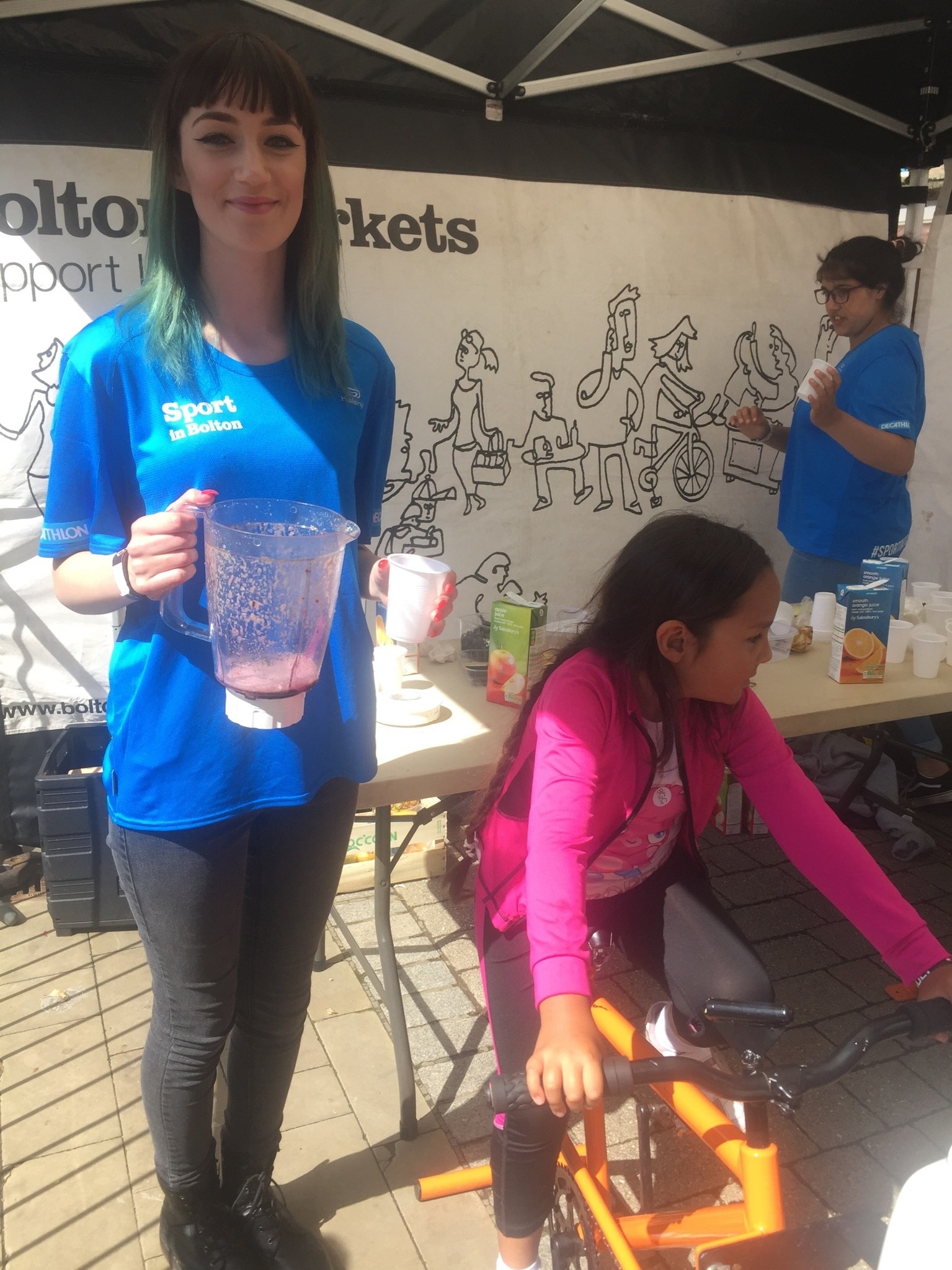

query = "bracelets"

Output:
[[750, 424, 775, 444]]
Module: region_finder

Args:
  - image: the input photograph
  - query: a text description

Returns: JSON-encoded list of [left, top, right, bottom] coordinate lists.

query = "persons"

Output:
[[34, 31, 458, 1270], [467, 509, 951, 1270], [727, 236, 951, 807]]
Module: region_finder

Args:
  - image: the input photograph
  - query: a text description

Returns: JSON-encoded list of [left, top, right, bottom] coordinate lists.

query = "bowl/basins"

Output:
[[457, 612, 491, 662], [458, 649, 489, 685], [768, 622, 799, 663]]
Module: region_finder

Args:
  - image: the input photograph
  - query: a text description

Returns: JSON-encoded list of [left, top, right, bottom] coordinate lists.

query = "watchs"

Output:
[[112, 546, 146, 600]]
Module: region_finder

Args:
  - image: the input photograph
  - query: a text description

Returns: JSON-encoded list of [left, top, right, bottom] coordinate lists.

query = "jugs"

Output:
[[159, 497, 362, 733]]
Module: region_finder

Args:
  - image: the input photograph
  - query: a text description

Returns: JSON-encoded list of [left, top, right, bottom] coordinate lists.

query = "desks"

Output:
[[313, 600, 952, 1143]]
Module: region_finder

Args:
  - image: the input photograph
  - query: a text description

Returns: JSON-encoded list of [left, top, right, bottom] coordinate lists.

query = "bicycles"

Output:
[[412, 923, 952, 1270]]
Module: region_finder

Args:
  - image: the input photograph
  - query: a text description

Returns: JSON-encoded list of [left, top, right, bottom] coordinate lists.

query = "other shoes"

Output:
[[646, 1000, 747, 1142], [888, 766, 952, 806]]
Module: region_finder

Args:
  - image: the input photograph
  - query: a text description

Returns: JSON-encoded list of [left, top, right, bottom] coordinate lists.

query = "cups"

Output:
[[384, 553, 452, 645], [912, 633, 948, 679], [796, 358, 839, 403], [908, 623, 948, 662], [886, 619, 914, 664], [931, 591, 952, 610], [373, 646, 408, 691], [945, 618, 952, 667], [911, 582, 941, 608], [809, 591, 836, 644], [924, 604, 952, 634], [770, 601, 794, 635]]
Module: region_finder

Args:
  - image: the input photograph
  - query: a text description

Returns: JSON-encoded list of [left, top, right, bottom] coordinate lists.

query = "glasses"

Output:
[[813, 283, 863, 304]]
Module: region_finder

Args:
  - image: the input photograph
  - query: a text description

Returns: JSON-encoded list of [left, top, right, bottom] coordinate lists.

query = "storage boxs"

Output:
[[34, 722, 138, 937]]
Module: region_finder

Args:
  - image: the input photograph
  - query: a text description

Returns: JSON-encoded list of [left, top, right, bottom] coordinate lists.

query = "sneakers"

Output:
[[220, 1157, 332, 1270], [155, 1164, 274, 1270]]
